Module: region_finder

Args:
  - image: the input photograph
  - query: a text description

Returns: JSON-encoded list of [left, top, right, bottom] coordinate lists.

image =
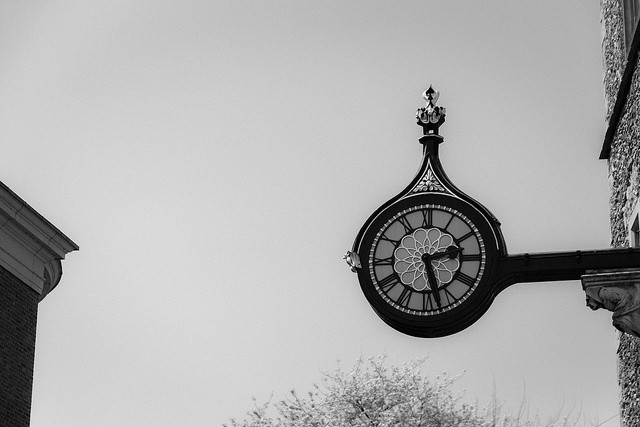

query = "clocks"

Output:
[[353, 195, 494, 337]]
